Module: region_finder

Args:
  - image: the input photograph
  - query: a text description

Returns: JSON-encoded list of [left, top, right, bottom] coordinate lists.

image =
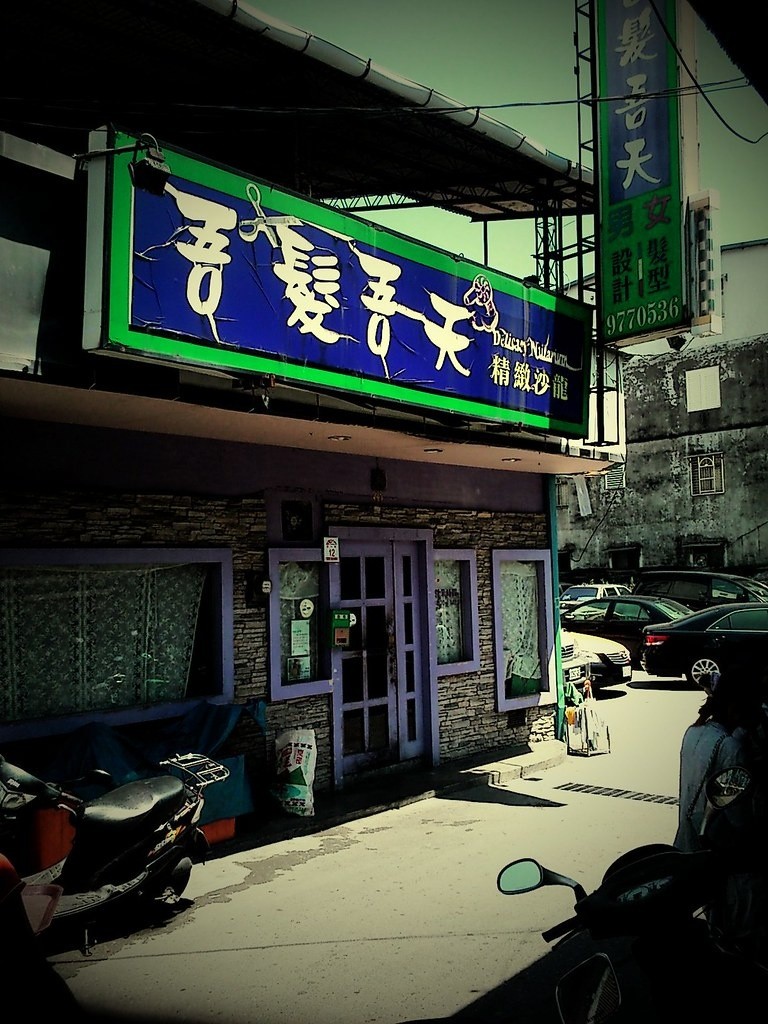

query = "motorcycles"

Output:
[[495, 763, 768, 1024], [0, 747, 233, 934]]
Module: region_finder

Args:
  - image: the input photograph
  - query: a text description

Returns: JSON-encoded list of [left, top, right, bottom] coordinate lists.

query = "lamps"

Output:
[[72, 141, 171, 198], [369, 458, 388, 502]]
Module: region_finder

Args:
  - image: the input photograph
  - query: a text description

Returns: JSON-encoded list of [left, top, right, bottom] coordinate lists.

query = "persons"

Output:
[[672, 667, 767, 869]]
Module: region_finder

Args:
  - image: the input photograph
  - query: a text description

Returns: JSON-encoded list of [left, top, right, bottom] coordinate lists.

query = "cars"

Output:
[[558, 583, 632, 616], [630, 571, 768, 616], [641, 602, 768, 694], [563, 628, 635, 694], [562, 594, 698, 662]]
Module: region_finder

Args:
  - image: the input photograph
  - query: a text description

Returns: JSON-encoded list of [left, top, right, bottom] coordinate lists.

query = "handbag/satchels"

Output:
[[691, 806, 729, 856]]
